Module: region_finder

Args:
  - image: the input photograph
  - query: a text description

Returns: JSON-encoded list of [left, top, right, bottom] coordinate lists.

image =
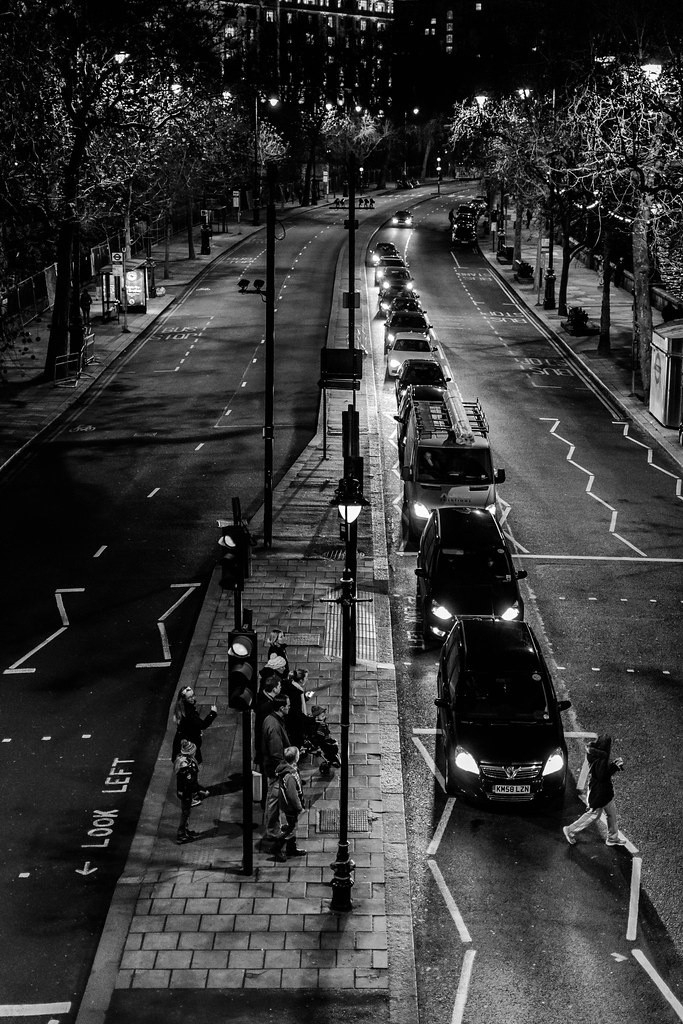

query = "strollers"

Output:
[[303, 735, 340, 778]]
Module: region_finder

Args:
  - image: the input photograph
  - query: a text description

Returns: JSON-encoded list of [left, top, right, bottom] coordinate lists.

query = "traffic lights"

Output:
[[218, 527, 246, 591], [227, 632, 258, 710]]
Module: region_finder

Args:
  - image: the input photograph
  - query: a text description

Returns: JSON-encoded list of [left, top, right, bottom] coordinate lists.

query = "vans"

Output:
[[403, 396, 507, 533]]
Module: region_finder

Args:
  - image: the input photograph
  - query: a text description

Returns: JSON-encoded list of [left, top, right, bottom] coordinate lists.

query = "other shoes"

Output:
[[200, 787, 205, 794], [190, 800, 200, 807], [288, 848, 307, 858], [187, 829, 196, 835], [176, 835, 194, 844], [606, 838, 626, 846], [270, 847, 286, 861], [562, 826, 576, 844], [265, 842, 272, 853]]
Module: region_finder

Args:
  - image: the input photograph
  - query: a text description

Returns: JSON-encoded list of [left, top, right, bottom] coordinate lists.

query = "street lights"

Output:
[[329, 472, 372, 913], [413, 106, 423, 175], [321, 86, 385, 349], [253, 90, 278, 226]]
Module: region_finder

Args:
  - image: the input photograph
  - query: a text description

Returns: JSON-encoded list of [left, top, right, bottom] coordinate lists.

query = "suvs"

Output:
[[433, 616, 571, 808], [413, 506, 529, 645]]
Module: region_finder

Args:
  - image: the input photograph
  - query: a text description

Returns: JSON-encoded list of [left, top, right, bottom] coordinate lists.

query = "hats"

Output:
[[265, 653, 286, 669], [180, 739, 196, 754], [311, 706, 327, 716]]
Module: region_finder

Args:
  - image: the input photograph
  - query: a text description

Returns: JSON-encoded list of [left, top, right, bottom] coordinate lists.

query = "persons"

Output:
[[264, 746, 306, 863], [174, 739, 200, 845], [171, 686, 218, 772], [562, 731, 628, 848], [79, 288, 93, 326], [526, 209, 532, 229], [251, 628, 338, 799]]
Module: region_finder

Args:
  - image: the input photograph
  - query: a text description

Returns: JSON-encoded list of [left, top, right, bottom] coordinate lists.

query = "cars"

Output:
[[387, 333, 438, 376], [370, 243, 434, 347], [391, 211, 414, 227], [450, 195, 487, 250], [394, 358, 452, 409]]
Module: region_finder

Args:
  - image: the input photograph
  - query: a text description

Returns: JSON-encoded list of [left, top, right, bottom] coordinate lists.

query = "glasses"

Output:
[[186, 694, 195, 700]]
